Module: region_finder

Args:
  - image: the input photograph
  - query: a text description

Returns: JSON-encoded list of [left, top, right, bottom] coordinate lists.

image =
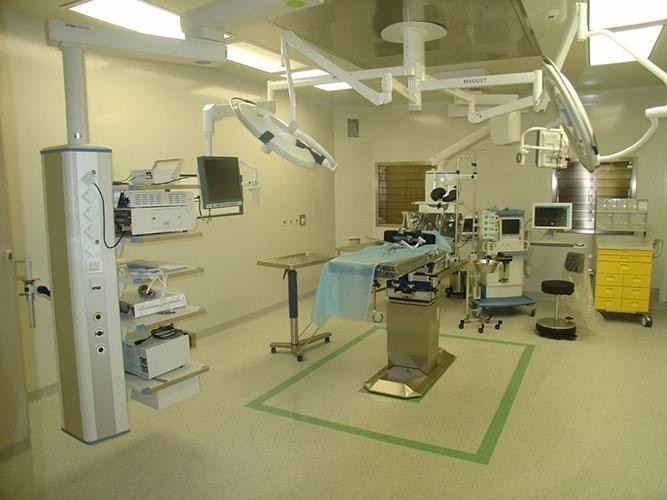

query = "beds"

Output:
[[327, 237, 455, 323]]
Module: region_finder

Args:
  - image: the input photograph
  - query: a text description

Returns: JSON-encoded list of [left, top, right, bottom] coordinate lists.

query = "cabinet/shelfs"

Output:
[[114, 227, 209, 394]]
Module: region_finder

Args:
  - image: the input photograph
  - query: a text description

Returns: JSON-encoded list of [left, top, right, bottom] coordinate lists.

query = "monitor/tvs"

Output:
[[532, 203, 572, 230], [500, 216, 524, 240], [197, 156, 243, 209], [461, 217, 478, 236]]
